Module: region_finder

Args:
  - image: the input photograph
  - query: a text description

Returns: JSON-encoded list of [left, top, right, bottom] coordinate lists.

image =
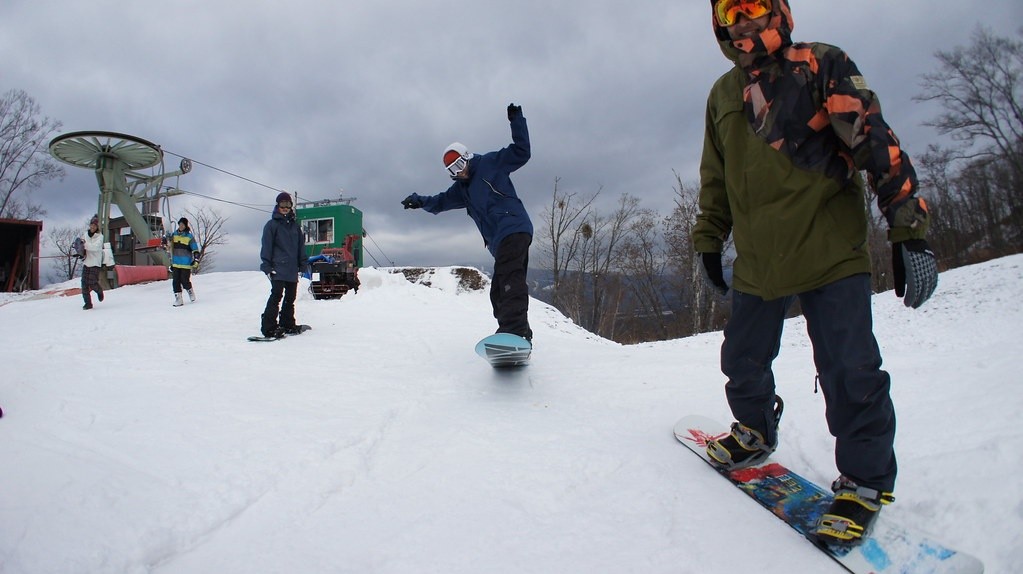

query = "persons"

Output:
[[260, 192, 308, 338], [401, 103, 534, 349], [692, 0, 938, 547], [161, 217, 200, 307], [80, 214, 104, 310]]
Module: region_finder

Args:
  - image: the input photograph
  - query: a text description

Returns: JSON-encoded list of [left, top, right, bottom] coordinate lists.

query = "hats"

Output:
[[90, 214, 99, 225], [276, 192, 292, 202], [178, 217, 188, 227]]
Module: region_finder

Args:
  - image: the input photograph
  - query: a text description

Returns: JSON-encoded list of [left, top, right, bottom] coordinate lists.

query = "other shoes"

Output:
[[260, 315, 300, 338], [83, 303, 93, 310], [98, 290, 104, 301]]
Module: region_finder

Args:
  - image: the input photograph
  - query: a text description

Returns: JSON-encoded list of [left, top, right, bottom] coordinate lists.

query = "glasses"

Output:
[[279, 201, 293, 208], [714, 0, 772, 27], [445, 156, 467, 176]]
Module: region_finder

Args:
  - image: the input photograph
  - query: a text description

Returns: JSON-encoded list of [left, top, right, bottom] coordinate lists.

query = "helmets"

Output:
[[443, 142, 474, 168]]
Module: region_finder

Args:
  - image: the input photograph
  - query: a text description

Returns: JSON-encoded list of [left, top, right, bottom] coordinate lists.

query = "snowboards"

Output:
[[248, 324, 312, 341], [474, 333, 531, 369], [672, 414, 985, 574]]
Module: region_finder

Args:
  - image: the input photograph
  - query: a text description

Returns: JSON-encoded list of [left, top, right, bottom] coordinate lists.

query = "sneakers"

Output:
[[187, 287, 196, 302], [172, 292, 183, 307], [708, 422, 775, 465], [818, 475, 882, 548]]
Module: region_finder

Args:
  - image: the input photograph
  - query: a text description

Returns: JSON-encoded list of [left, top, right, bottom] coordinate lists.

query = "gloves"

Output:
[[695, 251, 729, 296], [299, 261, 307, 273], [192, 261, 198, 269], [507, 102, 523, 121], [401, 195, 417, 210], [892, 238, 938, 309], [266, 270, 276, 282], [168, 265, 173, 272]]
